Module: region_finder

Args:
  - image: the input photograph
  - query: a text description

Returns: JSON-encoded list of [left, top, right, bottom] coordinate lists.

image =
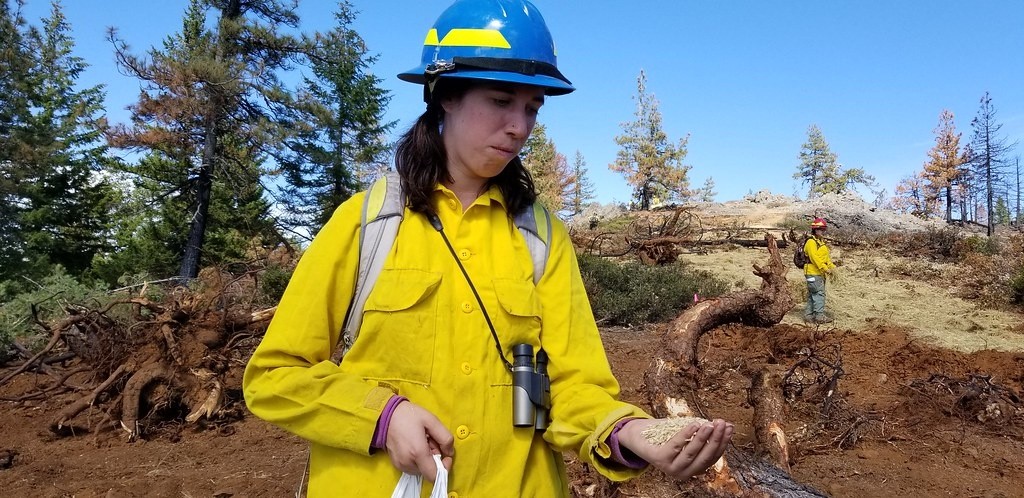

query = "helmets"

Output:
[[397, 0, 574, 96], [809, 218, 827, 229]]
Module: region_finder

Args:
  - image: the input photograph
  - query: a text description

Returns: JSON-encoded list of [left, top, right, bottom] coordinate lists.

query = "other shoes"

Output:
[[804, 315, 814, 323], [815, 314, 833, 324]]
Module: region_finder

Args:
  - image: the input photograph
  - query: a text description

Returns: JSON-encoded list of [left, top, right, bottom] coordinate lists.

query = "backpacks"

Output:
[[793, 235, 819, 269]]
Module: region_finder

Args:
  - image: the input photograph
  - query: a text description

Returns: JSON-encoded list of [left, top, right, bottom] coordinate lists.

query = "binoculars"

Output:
[[511, 343, 552, 431]]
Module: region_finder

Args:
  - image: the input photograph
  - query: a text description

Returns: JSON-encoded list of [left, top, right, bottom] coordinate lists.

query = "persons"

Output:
[[803, 217, 836, 323], [242, 0, 735, 498]]
